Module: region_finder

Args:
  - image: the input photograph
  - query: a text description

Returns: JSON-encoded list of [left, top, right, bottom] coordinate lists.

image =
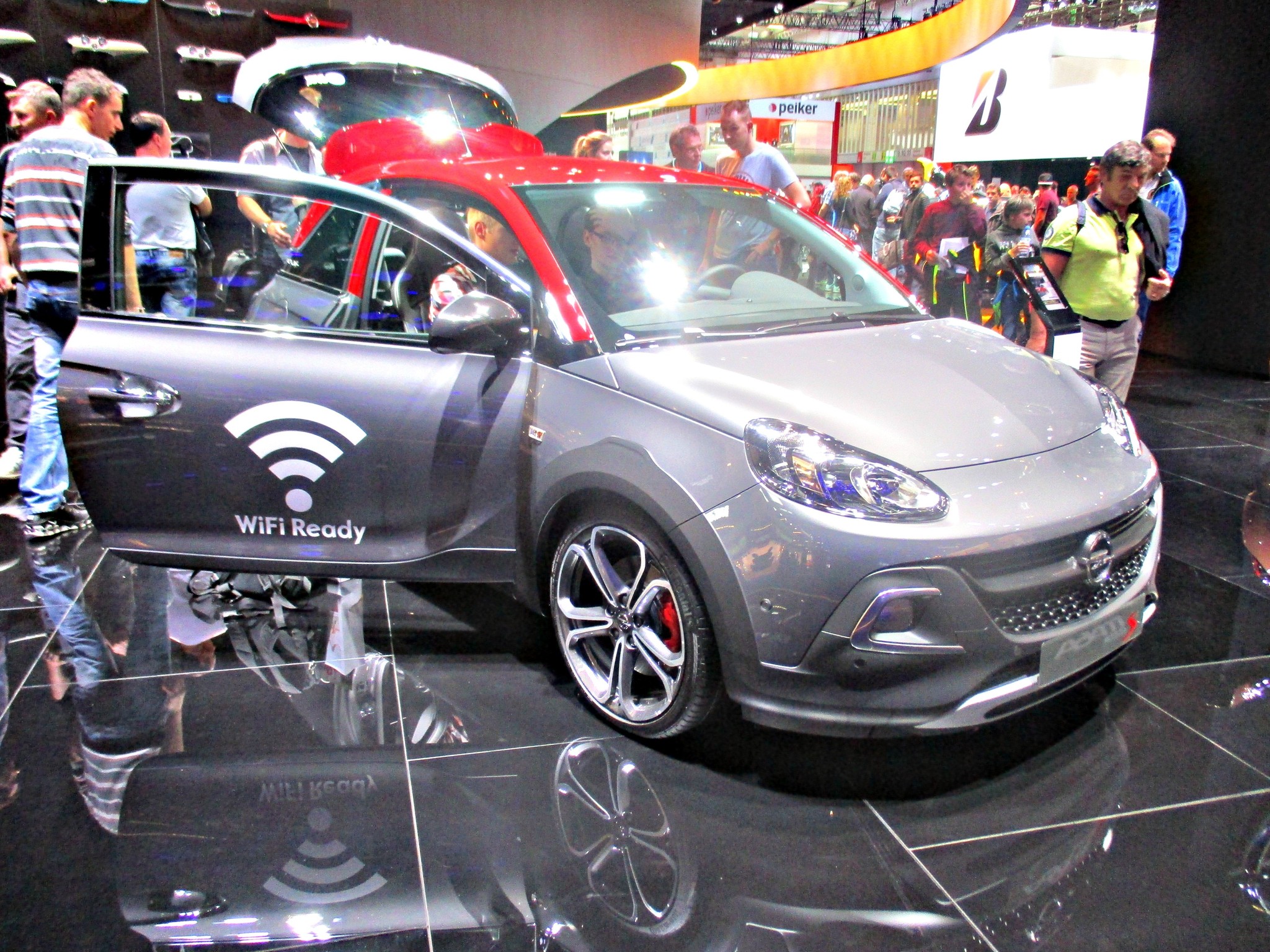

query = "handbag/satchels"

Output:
[[877, 240, 897, 271], [836, 228, 858, 241]]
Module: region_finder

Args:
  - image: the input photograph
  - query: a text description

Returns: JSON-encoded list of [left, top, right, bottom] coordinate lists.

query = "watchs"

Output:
[[261, 220, 273, 234]]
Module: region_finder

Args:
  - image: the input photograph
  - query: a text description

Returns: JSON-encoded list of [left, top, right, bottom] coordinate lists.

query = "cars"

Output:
[[112, 583, 1129, 951], [54, 34, 1167, 750]]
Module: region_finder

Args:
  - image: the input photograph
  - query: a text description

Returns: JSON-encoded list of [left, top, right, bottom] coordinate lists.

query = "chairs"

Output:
[[556, 206, 590, 274], [391, 207, 469, 334]]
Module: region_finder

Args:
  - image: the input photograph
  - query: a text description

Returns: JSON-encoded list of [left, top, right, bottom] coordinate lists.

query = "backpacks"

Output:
[[226, 602, 329, 691], [883, 182, 907, 217], [186, 570, 338, 600]]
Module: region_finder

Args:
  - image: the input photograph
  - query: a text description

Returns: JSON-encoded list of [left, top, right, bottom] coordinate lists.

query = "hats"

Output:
[[1038, 173, 1053, 185]]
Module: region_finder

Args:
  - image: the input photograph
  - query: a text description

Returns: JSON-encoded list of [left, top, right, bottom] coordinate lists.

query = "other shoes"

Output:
[[21, 489, 94, 537], [24, 523, 95, 567], [0, 447, 24, 479]]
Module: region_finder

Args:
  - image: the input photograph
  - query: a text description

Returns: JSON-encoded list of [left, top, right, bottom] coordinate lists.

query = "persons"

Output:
[[0, 64, 1185, 542], [28, 545, 469, 834], [1227, 463, 1269, 709]]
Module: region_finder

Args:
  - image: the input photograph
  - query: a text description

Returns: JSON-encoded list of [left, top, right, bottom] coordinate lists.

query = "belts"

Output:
[[1074, 313, 1129, 329]]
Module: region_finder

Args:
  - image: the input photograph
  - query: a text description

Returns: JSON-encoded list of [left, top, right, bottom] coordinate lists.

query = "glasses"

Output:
[[593, 231, 631, 247], [1114, 222, 1129, 253]]
